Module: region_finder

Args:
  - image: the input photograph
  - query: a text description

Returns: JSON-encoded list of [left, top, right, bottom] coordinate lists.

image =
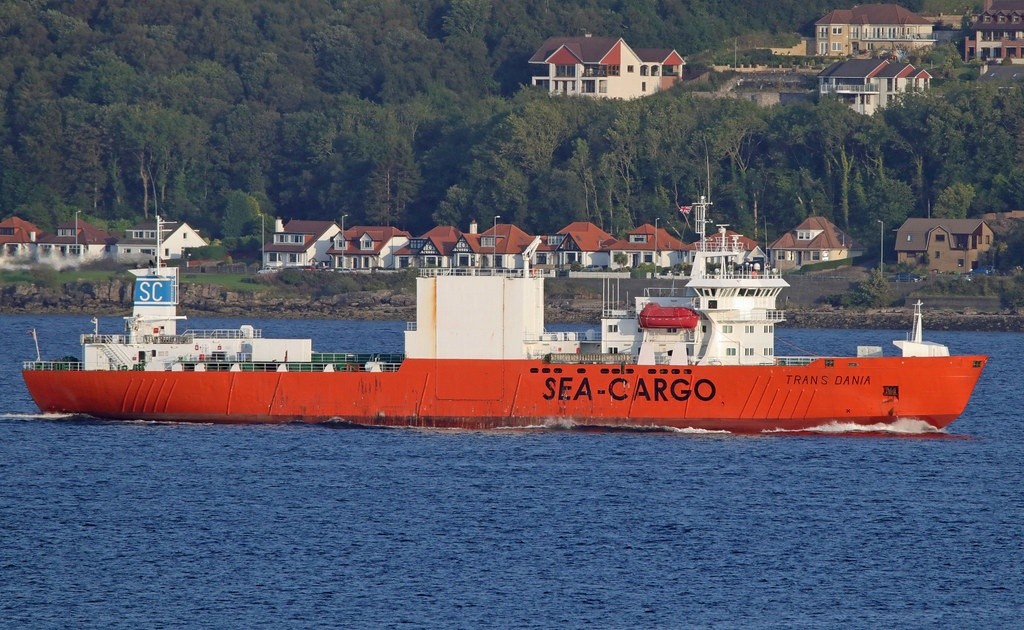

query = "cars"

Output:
[[971, 269, 993, 276], [257, 266, 277, 273], [895, 271, 921, 282], [282, 265, 352, 274]]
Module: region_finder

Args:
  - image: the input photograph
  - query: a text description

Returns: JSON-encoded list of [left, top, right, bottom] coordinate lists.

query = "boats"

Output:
[[639, 303, 699, 331], [18, 213, 991, 427]]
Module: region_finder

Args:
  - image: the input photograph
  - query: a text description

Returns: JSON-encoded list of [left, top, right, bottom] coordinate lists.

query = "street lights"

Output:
[[75, 210, 81, 255], [878, 219, 884, 278], [654, 218, 660, 277], [257, 214, 264, 271], [341, 214, 348, 269], [493, 215, 500, 270]]
